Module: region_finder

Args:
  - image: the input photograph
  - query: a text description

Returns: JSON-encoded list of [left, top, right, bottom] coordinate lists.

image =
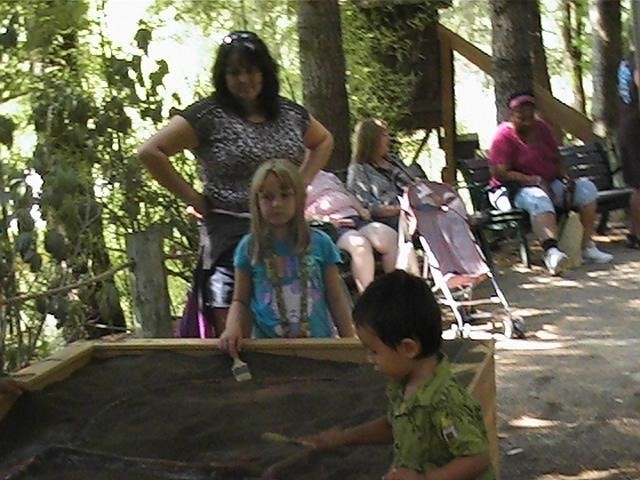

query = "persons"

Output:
[[219, 159, 356, 359], [298, 150, 400, 294], [344, 121, 419, 232], [139, 30, 336, 337], [614, 60, 640, 250], [489, 89, 614, 277], [295, 270, 496, 480]]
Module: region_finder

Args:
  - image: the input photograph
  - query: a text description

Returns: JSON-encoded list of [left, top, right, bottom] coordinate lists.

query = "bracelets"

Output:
[[415, 468, 428, 480]]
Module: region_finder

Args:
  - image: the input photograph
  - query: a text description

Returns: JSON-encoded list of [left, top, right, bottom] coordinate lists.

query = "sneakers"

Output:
[[582, 246, 612, 265], [542, 248, 569, 276]]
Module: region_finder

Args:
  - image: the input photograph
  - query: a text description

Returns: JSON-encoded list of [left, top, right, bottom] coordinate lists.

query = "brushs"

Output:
[[227, 347, 252, 383], [260, 429, 350, 456]]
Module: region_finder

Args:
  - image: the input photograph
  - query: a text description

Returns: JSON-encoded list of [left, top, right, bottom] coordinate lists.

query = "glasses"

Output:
[[221, 33, 253, 47]]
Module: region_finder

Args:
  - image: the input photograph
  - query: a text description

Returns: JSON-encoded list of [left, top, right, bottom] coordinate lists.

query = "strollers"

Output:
[[403, 181, 528, 343]]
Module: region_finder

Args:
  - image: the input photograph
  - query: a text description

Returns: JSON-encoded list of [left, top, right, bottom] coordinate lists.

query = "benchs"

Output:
[[452, 142, 635, 282], [331, 161, 425, 280]]
